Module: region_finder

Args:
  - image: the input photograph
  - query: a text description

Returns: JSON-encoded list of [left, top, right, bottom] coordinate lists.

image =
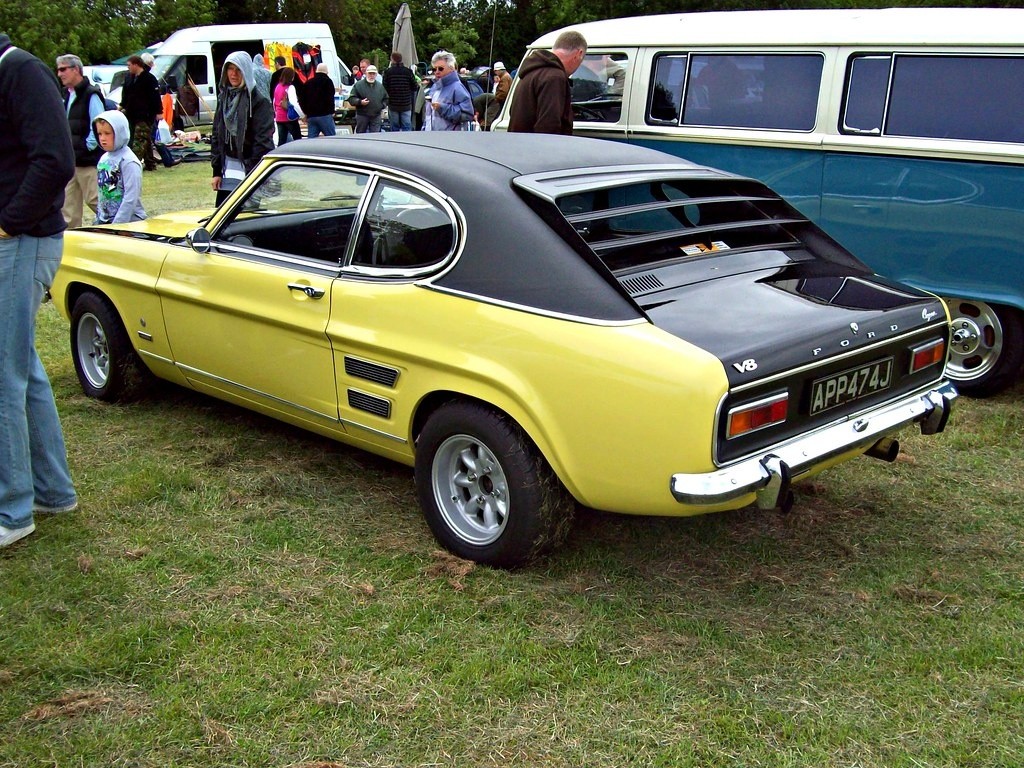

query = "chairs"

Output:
[[675, 56, 987, 139], [371, 219, 440, 267]]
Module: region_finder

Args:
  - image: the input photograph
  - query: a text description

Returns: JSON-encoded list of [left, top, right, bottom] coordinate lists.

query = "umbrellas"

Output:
[[472, 66, 491, 74], [389, 2, 419, 66]]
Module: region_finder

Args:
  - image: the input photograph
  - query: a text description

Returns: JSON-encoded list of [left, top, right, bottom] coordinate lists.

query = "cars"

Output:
[[39, 129, 960, 577], [104, 23, 343, 130], [421, 66, 492, 102]]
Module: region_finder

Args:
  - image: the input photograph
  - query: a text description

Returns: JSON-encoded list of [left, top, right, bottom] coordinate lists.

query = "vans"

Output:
[[334, 56, 357, 103], [81, 41, 162, 99], [488, 5, 1024, 398]]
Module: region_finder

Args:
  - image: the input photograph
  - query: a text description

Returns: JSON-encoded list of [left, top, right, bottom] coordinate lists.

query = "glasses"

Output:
[[431, 67, 448, 72], [56, 65, 75, 73]]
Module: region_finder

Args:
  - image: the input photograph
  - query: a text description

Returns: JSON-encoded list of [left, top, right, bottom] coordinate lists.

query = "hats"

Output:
[[316, 63, 329, 74], [365, 65, 378, 73], [494, 62, 506, 70]]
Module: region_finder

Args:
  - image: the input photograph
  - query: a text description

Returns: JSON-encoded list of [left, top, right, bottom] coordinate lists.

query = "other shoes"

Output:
[[174, 158, 181, 165], [32, 498, 77, 515], [0, 522, 36, 550]]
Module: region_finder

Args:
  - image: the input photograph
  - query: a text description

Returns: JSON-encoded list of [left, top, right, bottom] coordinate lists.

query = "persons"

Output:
[[460, 68, 466, 74], [695, 56, 750, 107], [348, 64, 388, 134], [117, 52, 154, 147], [353, 59, 383, 123], [269, 56, 304, 143], [571, 54, 625, 122], [57, 55, 107, 231], [421, 50, 474, 130], [492, 75, 501, 95], [472, 93, 499, 131], [382, 51, 420, 132], [0, 29, 79, 549], [507, 30, 587, 136], [494, 62, 513, 115], [122, 57, 164, 171], [273, 67, 307, 147], [252, 53, 272, 102], [92, 110, 147, 224], [303, 63, 336, 138], [210, 51, 282, 209]]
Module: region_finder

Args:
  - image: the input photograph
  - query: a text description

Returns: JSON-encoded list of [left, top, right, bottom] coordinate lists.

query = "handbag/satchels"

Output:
[[154, 118, 172, 146], [280, 96, 290, 109], [176, 71, 199, 116], [286, 101, 299, 122]]
